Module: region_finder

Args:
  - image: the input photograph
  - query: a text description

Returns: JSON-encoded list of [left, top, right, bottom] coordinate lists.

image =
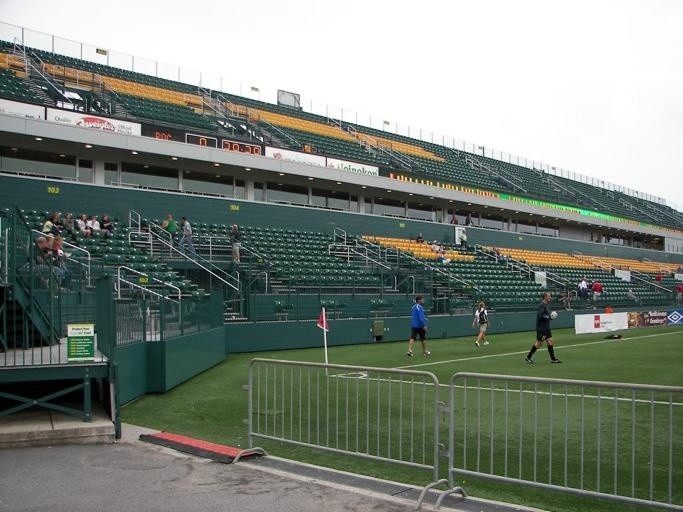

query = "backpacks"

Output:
[[478, 309, 488, 324]]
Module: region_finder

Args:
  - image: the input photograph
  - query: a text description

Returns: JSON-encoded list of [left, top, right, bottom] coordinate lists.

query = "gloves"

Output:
[[547, 316, 553, 320]]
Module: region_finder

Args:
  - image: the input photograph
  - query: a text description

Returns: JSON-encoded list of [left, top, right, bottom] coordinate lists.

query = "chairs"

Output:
[[0, 40, 683, 323]]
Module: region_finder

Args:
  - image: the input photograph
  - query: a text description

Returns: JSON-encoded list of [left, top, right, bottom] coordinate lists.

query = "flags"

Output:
[[316, 309, 330, 332]]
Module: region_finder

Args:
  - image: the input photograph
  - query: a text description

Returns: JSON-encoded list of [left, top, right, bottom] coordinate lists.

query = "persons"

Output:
[[557, 277, 603, 310], [417, 232, 424, 243], [162, 213, 178, 239], [228, 224, 241, 264], [178, 217, 195, 254], [407, 295, 431, 357], [605, 305, 613, 313], [466, 213, 474, 226], [34, 212, 115, 290], [450, 215, 458, 224], [525, 292, 561, 363], [459, 230, 467, 248], [430, 243, 452, 265], [472, 302, 490, 347]]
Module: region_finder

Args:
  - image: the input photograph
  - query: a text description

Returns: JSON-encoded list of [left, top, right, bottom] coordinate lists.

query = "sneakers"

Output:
[[423, 351, 432, 356], [550, 359, 563, 363], [525, 356, 534, 364], [406, 350, 415, 357], [475, 341, 489, 347]]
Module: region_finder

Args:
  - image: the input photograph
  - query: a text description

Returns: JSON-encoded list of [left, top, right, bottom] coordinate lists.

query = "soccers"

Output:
[[550, 311, 558, 319]]
